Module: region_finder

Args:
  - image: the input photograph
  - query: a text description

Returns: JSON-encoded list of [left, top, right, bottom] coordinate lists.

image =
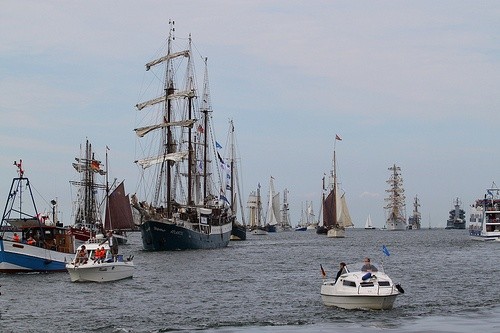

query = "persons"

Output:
[[361, 257, 379, 272], [11, 232, 48, 247], [334, 262, 351, 284], [159, 198, 230, 226], [71, 244, 108, 268]]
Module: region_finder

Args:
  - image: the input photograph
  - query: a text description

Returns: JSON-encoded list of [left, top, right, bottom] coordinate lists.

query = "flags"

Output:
[[195, 124, 235, 207], [335, 135, 342, 141]]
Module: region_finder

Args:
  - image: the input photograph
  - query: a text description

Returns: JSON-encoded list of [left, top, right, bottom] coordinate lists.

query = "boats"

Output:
[[3, 217, 47, 226], [320, 262, 401, 311], [468, 181, 500, 242], [445, 197, 466, 228], [295, 225, 307, 231], [0, 225, 76, 272], [66, 232, 135, 283]]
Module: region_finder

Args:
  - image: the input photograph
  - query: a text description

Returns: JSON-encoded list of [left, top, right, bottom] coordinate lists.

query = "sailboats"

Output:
[[383, 162, 406, 232], [281, 188, 290, 231], [63, 137, 105, 244], [302, 200, 315, 230], [216, 119, 247, 242], [316, 133, 353, 229], [132, 15, 233, 250], [99, 178, 137, 245], [407, 194, 421, 229], [245, 184, 261, 232], [260, 176, 280, 232], [365, 215, 375, 229]]
